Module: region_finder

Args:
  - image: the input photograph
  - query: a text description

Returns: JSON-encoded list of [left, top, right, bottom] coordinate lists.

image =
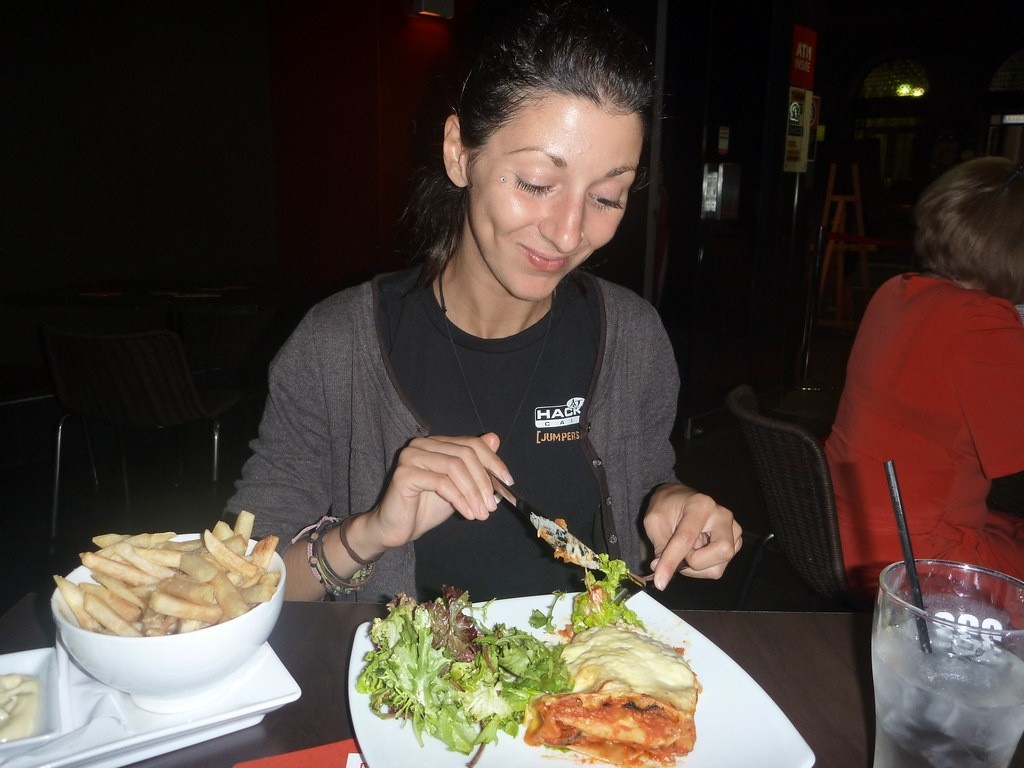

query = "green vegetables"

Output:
[[355, 552, 648, 751]]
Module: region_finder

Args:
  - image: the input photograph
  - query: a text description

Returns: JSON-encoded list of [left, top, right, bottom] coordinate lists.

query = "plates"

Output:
[[0, 639, 302, 767], [348, 590, 817, 767]]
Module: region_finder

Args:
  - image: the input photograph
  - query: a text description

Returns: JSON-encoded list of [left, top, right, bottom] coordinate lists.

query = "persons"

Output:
[[223, 0, 743, 609], [823, 157, 1024, 632]]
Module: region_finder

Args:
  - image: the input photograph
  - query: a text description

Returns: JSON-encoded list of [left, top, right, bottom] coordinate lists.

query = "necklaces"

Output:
[[439, 267, 555, 457]]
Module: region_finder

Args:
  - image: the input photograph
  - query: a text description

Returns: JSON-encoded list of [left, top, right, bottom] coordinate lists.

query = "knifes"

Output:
[[483, 467, 612, 576]]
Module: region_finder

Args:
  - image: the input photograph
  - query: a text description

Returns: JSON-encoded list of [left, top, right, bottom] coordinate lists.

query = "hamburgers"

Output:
[[524, 623, 702, 768]]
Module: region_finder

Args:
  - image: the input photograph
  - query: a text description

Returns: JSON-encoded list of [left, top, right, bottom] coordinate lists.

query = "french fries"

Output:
[[53, 510, 280, 635]]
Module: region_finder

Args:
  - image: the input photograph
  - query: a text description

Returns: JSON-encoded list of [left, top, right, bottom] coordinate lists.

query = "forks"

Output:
[[610, 559, 690, 608]]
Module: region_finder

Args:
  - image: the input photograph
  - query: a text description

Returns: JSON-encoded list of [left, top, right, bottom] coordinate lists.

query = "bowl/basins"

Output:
[[51, 534, 287, 713]]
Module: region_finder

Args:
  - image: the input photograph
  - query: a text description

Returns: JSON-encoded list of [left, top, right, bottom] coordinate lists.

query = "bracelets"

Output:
[[291, 516, 385, 597]]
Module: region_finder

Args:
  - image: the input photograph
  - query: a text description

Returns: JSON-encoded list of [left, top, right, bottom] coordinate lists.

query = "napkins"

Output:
[[0, 627, 132, 768]]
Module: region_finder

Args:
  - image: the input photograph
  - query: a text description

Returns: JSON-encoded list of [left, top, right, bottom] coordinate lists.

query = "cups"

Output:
[[871, 558, 1024, 768]]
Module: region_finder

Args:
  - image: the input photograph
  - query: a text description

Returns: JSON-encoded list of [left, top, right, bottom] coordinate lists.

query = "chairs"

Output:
[[726, 383, 881, 609], [41, 322, 266, 546], [0, 361, 103, 493]]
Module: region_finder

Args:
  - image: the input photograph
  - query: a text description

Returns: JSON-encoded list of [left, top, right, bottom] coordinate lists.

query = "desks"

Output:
[[0, 279, 277, 346], [1, 591, 1024, 768]]
